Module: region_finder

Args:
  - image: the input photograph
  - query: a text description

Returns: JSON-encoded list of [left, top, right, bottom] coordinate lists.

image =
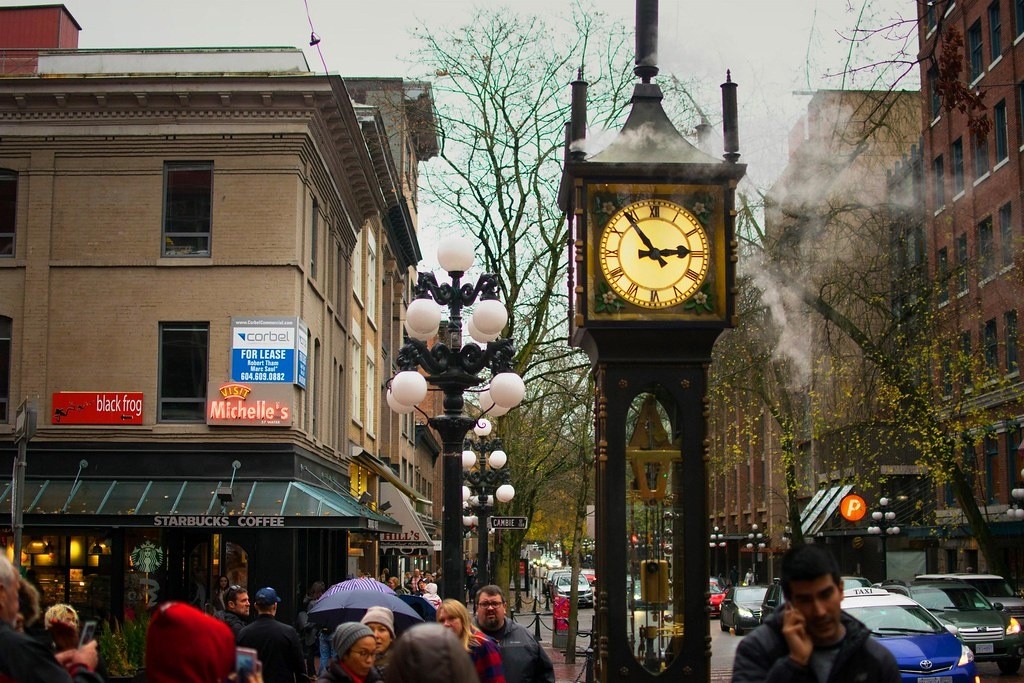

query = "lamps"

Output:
[[216, 486, 234, 507], [379, 500, 392, 512], [356, 491, 371, 505], [24, 536, 49, 554], [88, 537, 111, 555]]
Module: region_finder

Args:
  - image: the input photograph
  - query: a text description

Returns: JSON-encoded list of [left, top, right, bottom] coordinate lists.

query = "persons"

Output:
[[236, 588, 307, 683], [386, 624, 479, 683], [381, 567, 442, 610], [315, 622, 383, 683], [145, 603, 264, 683], [473, 586, 555, 683], [0, 554, 103, 683], [436, 599, 506, 683], [732, 543, 901, 683], [361, 606, 398, 675], [213, 585, 250, 638], [465, 562, 478, 603], [213, 576, 231, 611]]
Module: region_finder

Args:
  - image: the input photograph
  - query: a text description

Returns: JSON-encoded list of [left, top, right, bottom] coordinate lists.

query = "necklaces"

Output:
[[730, 565, 759, 586], [304, 582, 338, 680]]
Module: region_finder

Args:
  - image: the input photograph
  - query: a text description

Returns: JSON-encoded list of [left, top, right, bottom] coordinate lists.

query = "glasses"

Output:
[[226, 585, 241, 598], [477, 600, 505, 611]]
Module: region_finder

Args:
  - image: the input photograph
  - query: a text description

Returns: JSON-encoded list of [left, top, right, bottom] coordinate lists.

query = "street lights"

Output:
[[867, 498, 900, 581], [745, 524, 766, 585], [385, 234, 527, 610], [709, 526, 726, 577]]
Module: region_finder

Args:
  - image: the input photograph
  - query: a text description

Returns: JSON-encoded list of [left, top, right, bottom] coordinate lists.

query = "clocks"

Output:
[[583, 177, 732, 326]]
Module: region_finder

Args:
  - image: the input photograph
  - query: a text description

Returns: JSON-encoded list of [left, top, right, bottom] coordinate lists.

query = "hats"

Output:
[[332, 621, 377, 659], [360, 605, 396, 641], [256, 586, 281, 606]]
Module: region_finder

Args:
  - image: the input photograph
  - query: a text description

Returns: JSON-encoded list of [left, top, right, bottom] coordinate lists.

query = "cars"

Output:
[[839, 587, 981, 683], [709, 581, 769, 636], [531, 559, 669, 612]]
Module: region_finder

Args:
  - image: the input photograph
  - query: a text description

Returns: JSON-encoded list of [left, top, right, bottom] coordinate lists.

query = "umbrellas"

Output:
[[316, 579, 398, 604], [307, 589, 426, 632], [399, 594, 437, 622]]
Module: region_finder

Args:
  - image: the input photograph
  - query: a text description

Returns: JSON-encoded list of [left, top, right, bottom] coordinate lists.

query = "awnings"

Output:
[[381, 482, 435, 556]]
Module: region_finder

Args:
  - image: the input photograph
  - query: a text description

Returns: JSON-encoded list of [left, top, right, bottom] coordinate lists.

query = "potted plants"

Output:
[[100, 612, 146, 683]]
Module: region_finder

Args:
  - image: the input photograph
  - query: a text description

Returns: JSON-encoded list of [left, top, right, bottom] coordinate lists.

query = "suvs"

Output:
[[759, 576, 874, 625], [869, 571, 1024, 674]]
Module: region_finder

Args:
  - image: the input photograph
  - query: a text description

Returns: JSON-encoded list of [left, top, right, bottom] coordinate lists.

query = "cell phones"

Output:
[[234, 648, 258, 683], [786, 601, 805, 640], [78, 622, 95, 650]]
